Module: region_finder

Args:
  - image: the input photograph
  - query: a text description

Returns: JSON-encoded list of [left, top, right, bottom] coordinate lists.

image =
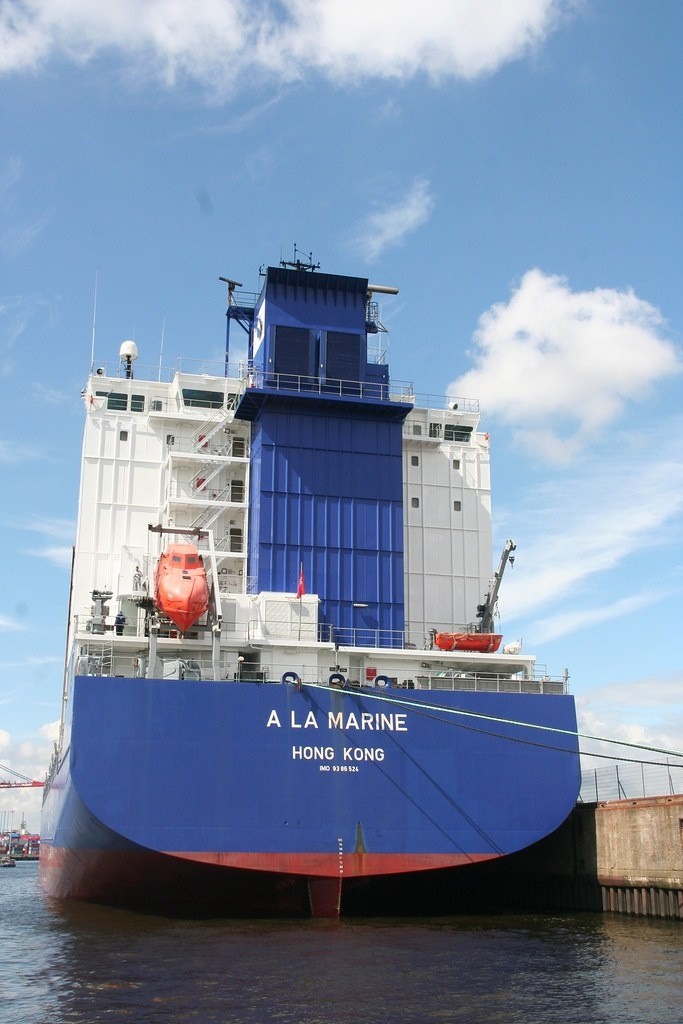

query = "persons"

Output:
[[133, 566, 144, 591], [115, 610, 128, 635]]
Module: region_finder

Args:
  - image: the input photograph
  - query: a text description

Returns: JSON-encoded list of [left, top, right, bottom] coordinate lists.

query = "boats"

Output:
[[0, 809, 37, 869]]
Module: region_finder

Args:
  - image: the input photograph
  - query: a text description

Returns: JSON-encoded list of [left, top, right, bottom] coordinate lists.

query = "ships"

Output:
[[39, 245, 583, 913]]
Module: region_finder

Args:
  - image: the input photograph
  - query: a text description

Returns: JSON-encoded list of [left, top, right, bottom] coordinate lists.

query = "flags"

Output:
[[295, 564, 305, 599]]
